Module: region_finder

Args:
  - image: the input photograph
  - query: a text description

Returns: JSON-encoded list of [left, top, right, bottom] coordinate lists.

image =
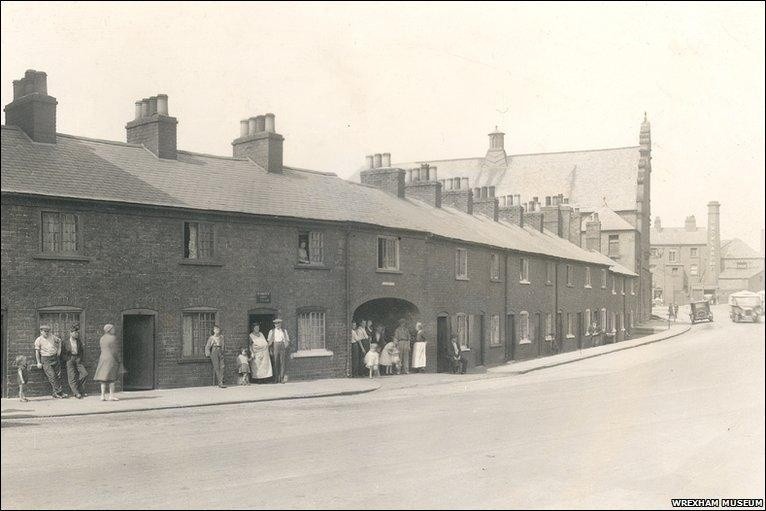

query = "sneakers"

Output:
[[101, 396, 119, 401], [21, 398, 28, 402], [53, 392, 87, 399], [219, 381, 225, 388]]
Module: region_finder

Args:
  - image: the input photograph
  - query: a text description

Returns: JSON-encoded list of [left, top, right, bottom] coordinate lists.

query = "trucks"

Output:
[[728, 290, 763, 322]]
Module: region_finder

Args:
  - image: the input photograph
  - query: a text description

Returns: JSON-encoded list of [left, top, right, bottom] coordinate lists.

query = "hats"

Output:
[[272, 319, 282, 323], [40, 325, 51, 330], [104, 324, 113, 333]]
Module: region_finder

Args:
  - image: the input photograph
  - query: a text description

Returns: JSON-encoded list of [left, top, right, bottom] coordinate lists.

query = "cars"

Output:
[[688, 299, 714, 323]]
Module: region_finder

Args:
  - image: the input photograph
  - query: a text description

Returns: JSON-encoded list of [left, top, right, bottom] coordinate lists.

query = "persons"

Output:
[[669, 303, 674, 316], [248, 324, 274, 385], [446, 333, 468, 375], [351, 317, 428, 377], [236, 348, 254, 386], [265, 319, 290, 384], [92, 323, 122, 402], [588, 320, 602, 349], [205, 326, 228, 388], [34, 326, 69, 399], [674, 303, 679, 319], [15, 355, 30, 404], [61, 325, 88, 399]]
